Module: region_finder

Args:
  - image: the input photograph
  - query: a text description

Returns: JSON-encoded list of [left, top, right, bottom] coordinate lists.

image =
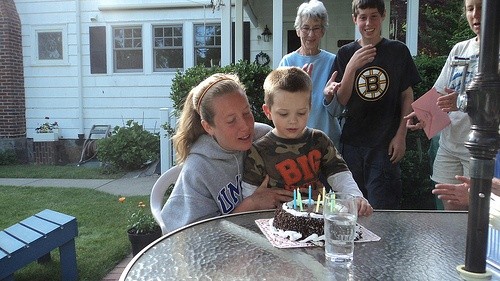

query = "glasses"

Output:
[[299, 26, 322, 33]]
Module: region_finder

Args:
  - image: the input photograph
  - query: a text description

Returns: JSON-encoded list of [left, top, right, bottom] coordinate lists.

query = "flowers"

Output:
[[36, 116, 59, 133], [128, 201, 161, 235]]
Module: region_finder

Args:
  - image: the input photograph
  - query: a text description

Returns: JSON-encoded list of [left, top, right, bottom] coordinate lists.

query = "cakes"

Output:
[[269, 200, 361, 245]]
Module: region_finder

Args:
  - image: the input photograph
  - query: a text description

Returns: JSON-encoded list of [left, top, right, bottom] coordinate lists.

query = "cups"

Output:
[[324, 192, 357, 264]]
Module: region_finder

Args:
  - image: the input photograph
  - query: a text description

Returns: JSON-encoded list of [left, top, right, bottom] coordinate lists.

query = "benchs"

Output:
[[0, 210, 78, 281]]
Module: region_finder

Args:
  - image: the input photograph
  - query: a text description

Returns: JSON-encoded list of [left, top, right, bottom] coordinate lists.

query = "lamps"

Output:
[[261, 24, 271, 42]]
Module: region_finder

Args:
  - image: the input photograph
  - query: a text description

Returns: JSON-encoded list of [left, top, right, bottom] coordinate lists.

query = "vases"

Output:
[[34, 130, 59, 141], [128, 226, 161, 256]]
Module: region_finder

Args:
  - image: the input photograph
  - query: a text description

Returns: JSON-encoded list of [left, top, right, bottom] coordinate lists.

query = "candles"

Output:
[[293, 185, 336, 213]]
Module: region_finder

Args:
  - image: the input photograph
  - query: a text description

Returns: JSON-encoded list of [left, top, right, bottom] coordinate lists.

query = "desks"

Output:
[[119, 209, 468, 281]]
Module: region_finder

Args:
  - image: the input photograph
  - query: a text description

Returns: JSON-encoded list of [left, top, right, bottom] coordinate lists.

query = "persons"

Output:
[[403, 0, 500, 211], [325, 0, 423, 210], [161, 73, 294, 232], [432, 175, 500, 209], [241, 66, 374, 217], [278, 0, 344, 151]]
